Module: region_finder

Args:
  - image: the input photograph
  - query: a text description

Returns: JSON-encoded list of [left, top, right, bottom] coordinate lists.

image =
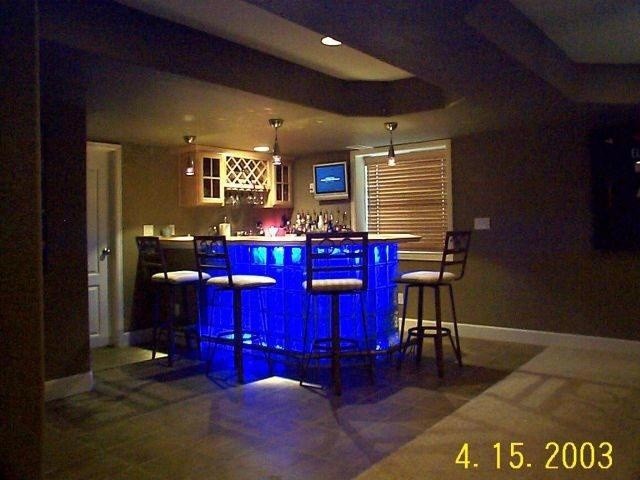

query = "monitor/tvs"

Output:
[[312, 161, 349, 202]]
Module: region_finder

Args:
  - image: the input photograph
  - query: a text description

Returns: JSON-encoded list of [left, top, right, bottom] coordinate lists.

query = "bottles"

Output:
[[213, 226, 218, 237], [295, 207, 348, 234]]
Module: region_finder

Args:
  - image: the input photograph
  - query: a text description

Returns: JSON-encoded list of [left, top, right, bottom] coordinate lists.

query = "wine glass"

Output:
[[225, 188, 265, 206]]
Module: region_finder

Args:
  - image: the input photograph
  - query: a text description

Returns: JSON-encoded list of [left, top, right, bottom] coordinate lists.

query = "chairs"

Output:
[[393, 228, 471, 379], [193, 234, 277, 385], [135, 235, 211, 368], [299, 230, 369, 396]]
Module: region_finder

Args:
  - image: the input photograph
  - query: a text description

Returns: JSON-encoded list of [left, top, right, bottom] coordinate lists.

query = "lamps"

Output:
[[384, 122, 398, 167], [183, 135, 196, 176], [268, 118, 285, 167]]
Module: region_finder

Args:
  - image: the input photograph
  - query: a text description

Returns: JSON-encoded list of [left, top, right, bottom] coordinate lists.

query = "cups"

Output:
[[168, 225, 176, 235]]
